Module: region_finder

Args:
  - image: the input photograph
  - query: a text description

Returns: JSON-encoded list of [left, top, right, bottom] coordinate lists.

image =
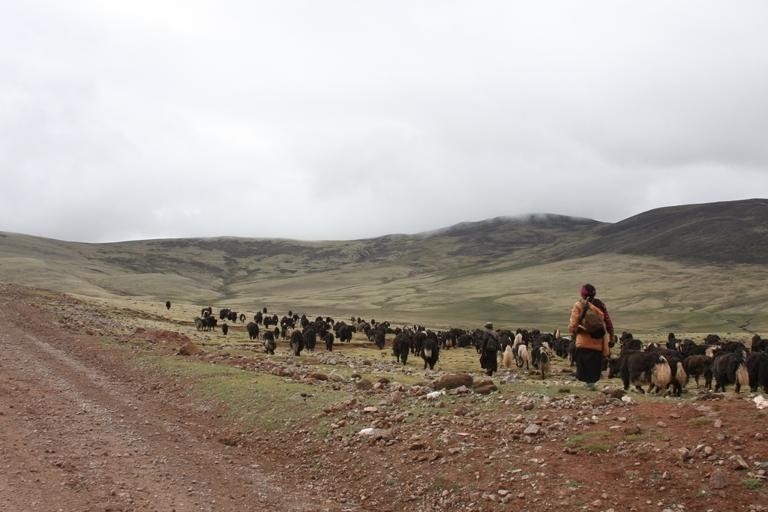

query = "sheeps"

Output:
[[193, 307, 246, 336], [166, 300, 171, 311], [396, 326, 571, 379], [609, 331, 767, 394], [246, 311, 394, 357]]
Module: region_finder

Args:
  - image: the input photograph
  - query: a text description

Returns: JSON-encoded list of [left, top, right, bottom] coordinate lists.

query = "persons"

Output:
[[512, 328, 525, 351], [568, 284, 615, 391], [480, 322, 502, 376]]
